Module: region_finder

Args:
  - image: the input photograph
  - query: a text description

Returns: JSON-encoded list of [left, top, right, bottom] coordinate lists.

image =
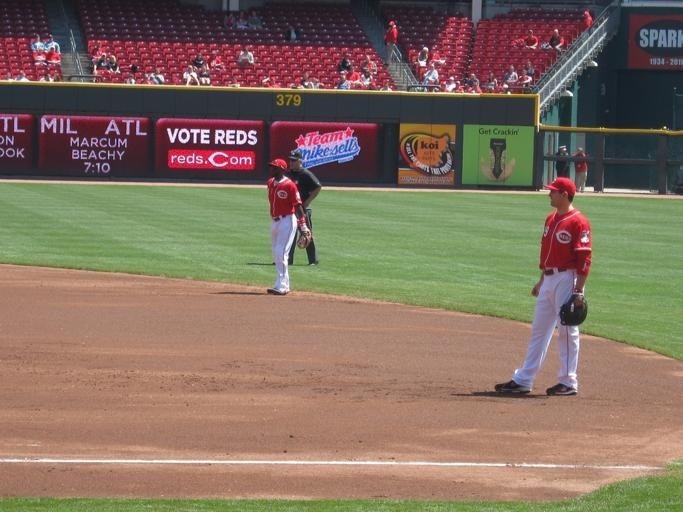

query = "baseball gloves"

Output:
[[297, 230, 311, 248], [559, 294, 587, 326]]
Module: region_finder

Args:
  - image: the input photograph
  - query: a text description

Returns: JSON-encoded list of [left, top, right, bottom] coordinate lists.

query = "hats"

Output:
[[266, 158, 288, 172], [287, 151, 302, 160], [558, 144, 567, 149], [543, 177, 576, 197]]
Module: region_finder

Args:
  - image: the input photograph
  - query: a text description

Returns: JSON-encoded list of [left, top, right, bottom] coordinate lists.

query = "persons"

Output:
[[14, 33, 60, 82], [261, 76, 280, 87], [178, 49, 222, 85], [542, 28, 565, 55], [288, 53, 393, 91], [263, 158, 312, 296], [569, 147, 587, 194], [285, 25, 299, 39], [271, 152, 321, 267], [553, 144, 569, 178], [124, 64, 165, 85], [88, 39, 120, 75], [417, 46, 534, 94], [226, 77, 241, 87], [523, 28, 539, 51], [382, 20, 398, 65], [221, 8, 263, 32], [492, 175, 593, 400], [583, 9, 593, 28], [236, 46, 254, 62]]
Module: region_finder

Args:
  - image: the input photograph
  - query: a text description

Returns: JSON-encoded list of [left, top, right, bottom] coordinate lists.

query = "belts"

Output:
[[543, 267, 567, 276]]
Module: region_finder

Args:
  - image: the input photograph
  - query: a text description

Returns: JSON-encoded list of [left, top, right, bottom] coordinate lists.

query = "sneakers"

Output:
[[273, 260, 320, 265], [546, 383, 578, 396], [494, 379, 533, 394], [267, 287, 290, 295]]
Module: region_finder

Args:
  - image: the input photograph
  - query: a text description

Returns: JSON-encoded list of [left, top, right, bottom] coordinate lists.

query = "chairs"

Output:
[[75, 2, 397, 91], [385, 3, 589, 93], [1, 1, 64, 82]]
